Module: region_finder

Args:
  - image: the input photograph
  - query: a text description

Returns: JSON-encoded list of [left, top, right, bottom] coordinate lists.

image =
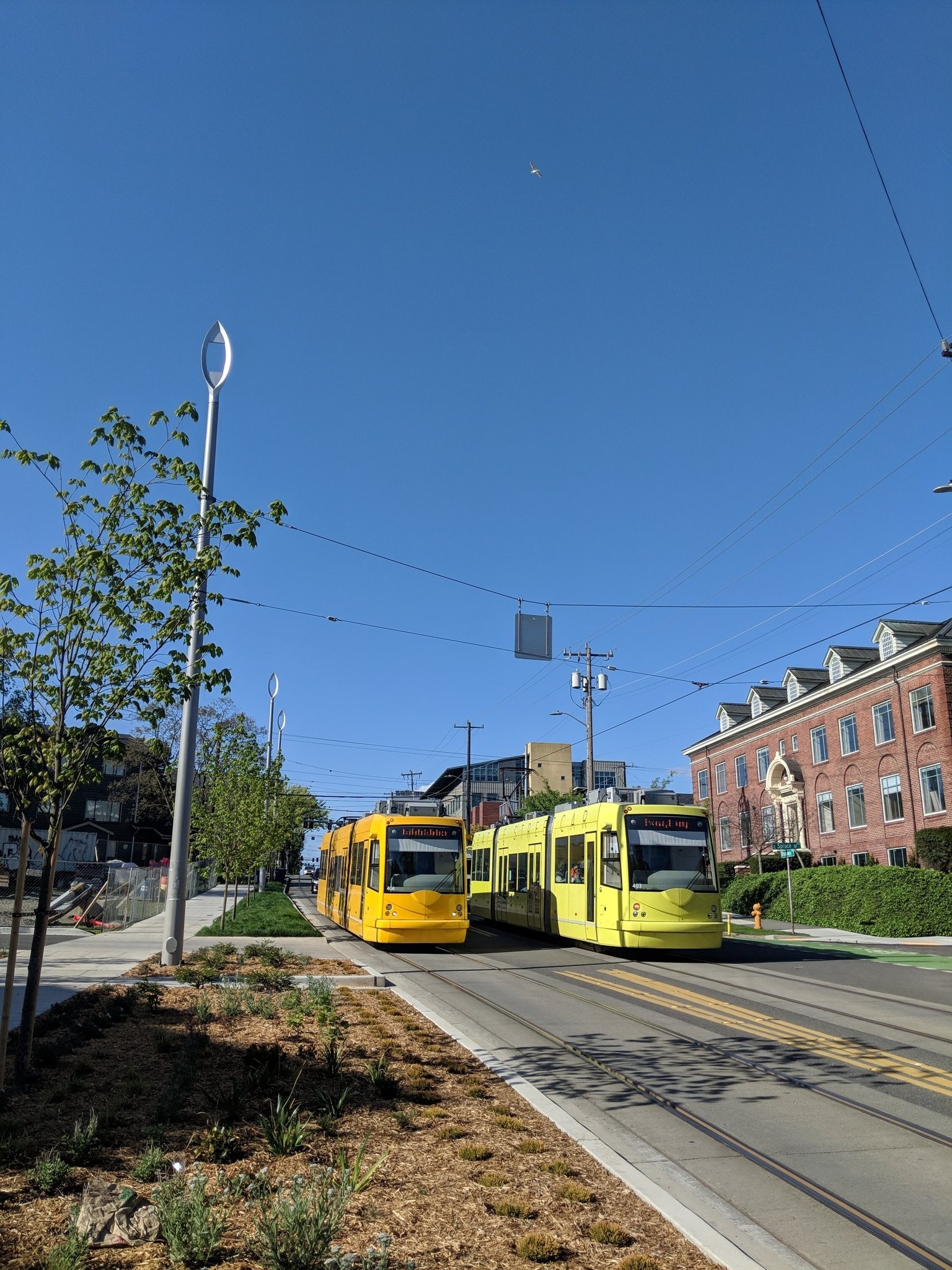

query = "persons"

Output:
[[570, 862, 583, 883], [554, 860, 566, 882]]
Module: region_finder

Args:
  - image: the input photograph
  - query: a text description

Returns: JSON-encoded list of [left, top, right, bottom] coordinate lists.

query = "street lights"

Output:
[[549, 710, 594, 791], [258, 670, 286, 892], [161, 321, 235, 968]]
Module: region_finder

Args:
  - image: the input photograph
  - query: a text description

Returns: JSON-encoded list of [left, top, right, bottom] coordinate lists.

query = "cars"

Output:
[[303, 866, 314, 875], [310, 868, 320, 894]]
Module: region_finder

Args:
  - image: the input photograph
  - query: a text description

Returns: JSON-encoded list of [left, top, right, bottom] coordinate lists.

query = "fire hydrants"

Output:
[[750, 902, 763, 929]]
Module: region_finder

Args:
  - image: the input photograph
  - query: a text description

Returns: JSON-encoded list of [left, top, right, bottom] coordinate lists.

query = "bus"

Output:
[[316, 812, 471, 950], [469, 766, 724, 953]]
